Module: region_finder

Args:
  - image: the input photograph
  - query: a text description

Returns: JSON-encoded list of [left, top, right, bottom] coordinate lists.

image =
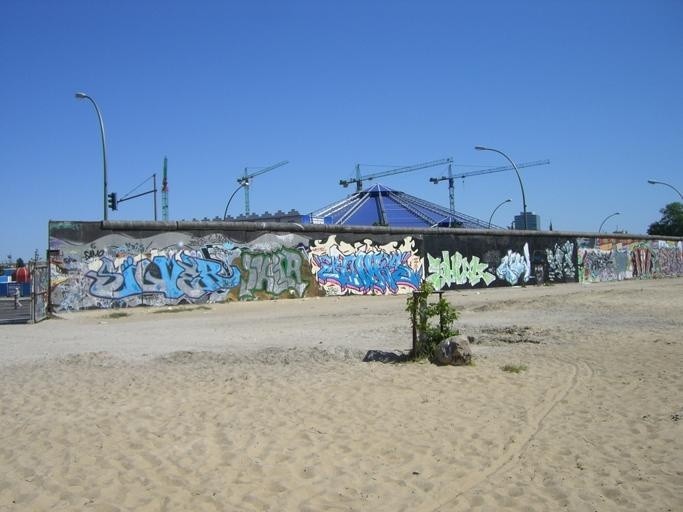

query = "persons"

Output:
[[11, 287, 23, 309]]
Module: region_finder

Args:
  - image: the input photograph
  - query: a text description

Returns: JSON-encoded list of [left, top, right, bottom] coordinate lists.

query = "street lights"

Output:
[[475, 146, 528, 228], [487, 198, 513, 228], [599, 213, 619, 232], [648, 180, 682, 199], [76, 92, 108, 221], [223, 183, 248, 219]]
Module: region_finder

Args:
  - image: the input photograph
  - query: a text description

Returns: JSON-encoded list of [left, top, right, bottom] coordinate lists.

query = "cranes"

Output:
[[239, 161, 289, 215], [340, 156, 454, 194], [161, 156, 168, 220], [429, 160, 552, 224]]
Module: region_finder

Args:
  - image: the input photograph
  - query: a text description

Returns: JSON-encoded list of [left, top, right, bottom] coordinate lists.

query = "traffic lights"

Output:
[[109, 192, 117, 210]]
